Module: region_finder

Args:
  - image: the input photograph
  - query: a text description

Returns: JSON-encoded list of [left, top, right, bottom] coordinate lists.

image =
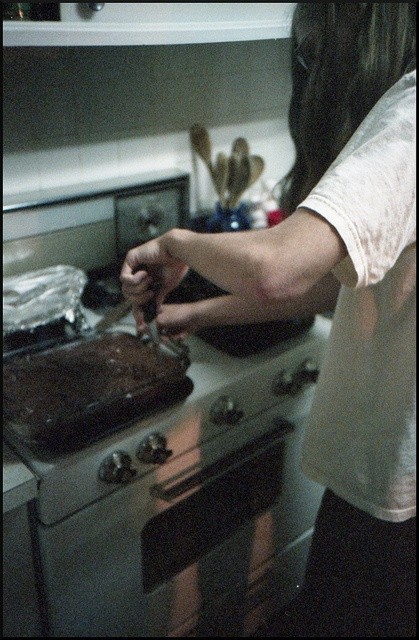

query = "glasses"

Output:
[[294, 21, 320, 73]]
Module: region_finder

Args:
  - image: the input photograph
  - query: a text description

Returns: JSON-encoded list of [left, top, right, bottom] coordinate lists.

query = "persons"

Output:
[[119, 1, 418, 637]]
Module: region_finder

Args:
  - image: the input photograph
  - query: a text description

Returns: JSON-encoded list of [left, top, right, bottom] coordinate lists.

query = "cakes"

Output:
[[1, 331, 180, 423]]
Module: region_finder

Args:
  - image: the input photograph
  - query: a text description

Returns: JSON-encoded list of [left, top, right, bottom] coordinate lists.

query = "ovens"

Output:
[[34, 380, 328, 639]]
